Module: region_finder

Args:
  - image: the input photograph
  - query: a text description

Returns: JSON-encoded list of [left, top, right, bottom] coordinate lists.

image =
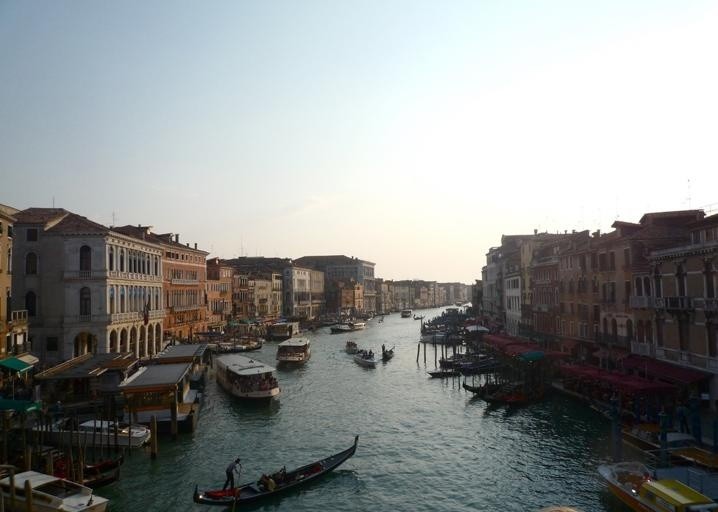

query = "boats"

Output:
[[0, 462, 111, 512], [616, 416, 717, 474], [420, 301, 551, 412], [275, 361, 305, 373], [594, 457, 717, 512], [187, 309, 420, 358], [274, 335, 311, 364], [354, 349, 378, 367], [18, 441, 125, 476], [534, 506, 583, 512], [18, 457, 121, 492], [214, 353, 279, 408], [189, 432, 360, 506], [28, 416, 150, 451]]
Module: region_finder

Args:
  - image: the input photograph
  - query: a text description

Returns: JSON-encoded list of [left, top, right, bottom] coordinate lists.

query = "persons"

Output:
[[676, 400, 692, 433], [592, 382, 646, 415], [347, 341, 386, 359], [222, 458, 240, 493], [238, 375, 278, 392]]
